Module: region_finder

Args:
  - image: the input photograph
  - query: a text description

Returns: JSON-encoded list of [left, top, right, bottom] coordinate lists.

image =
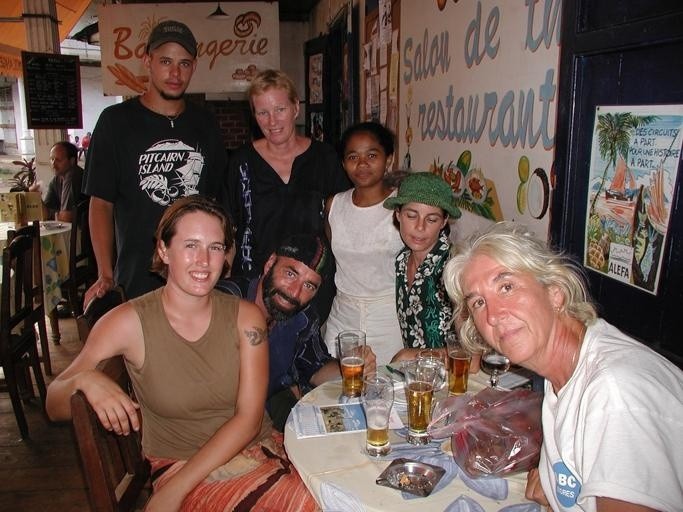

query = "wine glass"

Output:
[[480, 347, 514, 391]]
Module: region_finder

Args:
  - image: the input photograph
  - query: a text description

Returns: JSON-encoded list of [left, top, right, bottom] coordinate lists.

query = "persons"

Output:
[[216, 223, 377, 439], [319, 120, 407, 368], [382, 168, 486, 374], [79, 131, 90, 161], [223, 68, 355, 330], [81, 20, 234, 312], [26, 138, 93, 321], [439, 218, 683, 512], [70, 136, 82, 161], [41, 193, 322, 511]]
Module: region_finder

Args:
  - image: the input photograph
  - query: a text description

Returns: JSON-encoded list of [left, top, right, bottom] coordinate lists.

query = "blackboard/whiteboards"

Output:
[[21, 50, 83, 129]]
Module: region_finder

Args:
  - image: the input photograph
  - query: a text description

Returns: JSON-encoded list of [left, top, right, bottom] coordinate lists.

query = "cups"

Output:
[[361, 372, 394, 457], [403, 331, 475, 444], [336, 331, 365, 404]]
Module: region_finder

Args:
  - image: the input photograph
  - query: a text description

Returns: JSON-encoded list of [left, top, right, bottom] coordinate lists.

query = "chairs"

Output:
[[71, 354, 151, 512], [0, 199, 98, 441], [77, 284, 128, 345]]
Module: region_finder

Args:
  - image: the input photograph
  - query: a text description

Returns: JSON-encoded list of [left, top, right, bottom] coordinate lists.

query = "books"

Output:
[[320, 397, 405, 436], [291, 404, 326, 439]]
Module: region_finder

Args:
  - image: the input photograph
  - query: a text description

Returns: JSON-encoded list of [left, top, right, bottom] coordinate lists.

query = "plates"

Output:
[[42, 221, 63, 228], [443, 163, 465, 196], [465, 170, 489, 205]]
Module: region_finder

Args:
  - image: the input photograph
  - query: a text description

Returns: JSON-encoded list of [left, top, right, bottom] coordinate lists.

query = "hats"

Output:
[[382, 170, 462, 219], [146, 19, 197, 58], [250, 229, 335, 280]]
